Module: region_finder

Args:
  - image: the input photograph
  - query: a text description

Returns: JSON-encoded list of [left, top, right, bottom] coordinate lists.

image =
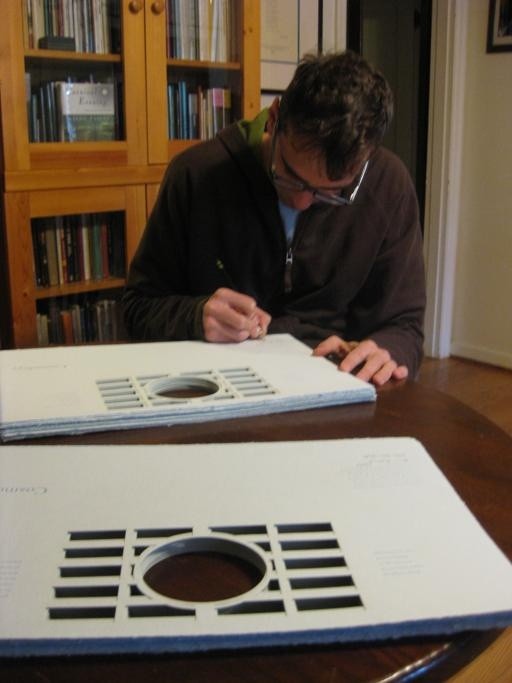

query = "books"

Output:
[[35, 298, 115, 347], [168, 75, 232, 141], [28, 1, 109, 53], [26, 73, 120, 143], [167, 0, 230, 62], [33, 213, 110, 288]]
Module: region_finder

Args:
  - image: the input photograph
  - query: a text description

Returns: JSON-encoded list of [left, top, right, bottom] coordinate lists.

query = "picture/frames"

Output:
[[484, 0, 512, 52]]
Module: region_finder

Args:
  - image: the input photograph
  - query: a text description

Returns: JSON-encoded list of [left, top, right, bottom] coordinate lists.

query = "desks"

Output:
[[0, 332, 511, 681]]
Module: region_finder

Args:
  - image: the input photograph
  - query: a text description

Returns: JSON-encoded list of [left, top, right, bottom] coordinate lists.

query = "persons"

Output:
[[116, 47, 431, 390]]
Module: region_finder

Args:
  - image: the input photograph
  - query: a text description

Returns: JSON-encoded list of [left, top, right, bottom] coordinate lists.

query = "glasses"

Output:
[[267, 116, 369, 206]]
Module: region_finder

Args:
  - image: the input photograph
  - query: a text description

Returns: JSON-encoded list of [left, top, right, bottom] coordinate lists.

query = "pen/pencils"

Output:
[[216, 260, 272, 343]]
